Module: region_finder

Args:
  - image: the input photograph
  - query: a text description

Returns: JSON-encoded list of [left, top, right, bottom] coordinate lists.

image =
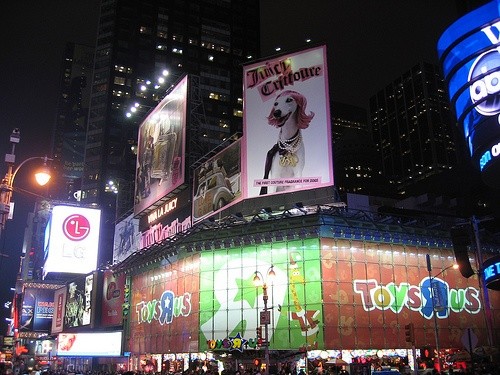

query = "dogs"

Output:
[[266, 95, 305, 194]]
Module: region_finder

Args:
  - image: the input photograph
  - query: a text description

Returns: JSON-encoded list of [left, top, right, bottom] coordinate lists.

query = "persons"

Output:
[[64, 281, 86, 328], [28, 352, 475, 375]]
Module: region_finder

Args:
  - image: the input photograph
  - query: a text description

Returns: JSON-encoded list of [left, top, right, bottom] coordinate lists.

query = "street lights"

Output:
[[249, 265, 279, 375], [425, 253, 461, 375], [0, 155, 58, 375]]
